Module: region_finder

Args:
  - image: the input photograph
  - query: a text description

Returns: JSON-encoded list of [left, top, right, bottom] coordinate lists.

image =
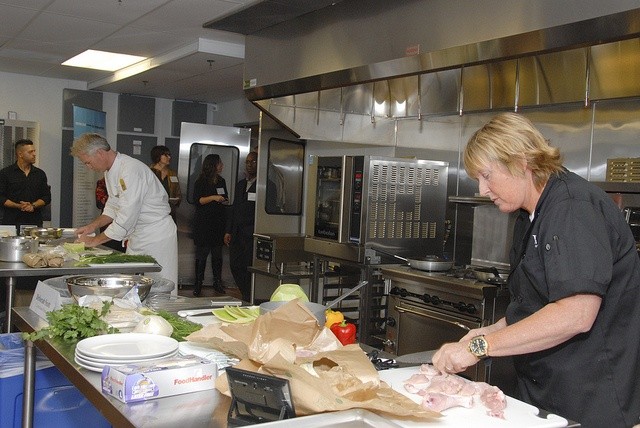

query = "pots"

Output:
[[259, 281, 369, 329], [0, 235, 39, 262], [371, 246, 455, 272], [472, 266, 509, 284]]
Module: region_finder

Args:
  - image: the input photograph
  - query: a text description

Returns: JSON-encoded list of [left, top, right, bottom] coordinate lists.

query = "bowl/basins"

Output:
[[64, 274, 155, 306]]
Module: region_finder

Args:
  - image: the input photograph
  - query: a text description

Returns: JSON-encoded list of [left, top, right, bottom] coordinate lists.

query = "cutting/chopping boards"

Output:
[[377, 365, 569, 428], [47, 233, 93, 247]]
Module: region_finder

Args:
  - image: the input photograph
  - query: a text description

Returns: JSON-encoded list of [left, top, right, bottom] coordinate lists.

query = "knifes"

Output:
[[395, 350, 439, 366]]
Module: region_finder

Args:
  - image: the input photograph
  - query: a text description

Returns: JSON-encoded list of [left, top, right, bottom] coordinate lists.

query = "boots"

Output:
[[193, 260, 205, 297], [212, 259, 226, 294]]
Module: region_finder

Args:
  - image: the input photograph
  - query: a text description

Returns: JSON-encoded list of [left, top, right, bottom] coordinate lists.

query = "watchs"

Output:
[[467, 334, 488, 361]]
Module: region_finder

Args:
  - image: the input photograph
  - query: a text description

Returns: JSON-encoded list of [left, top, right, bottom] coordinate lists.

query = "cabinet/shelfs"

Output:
[[0, 118, 40, 170]]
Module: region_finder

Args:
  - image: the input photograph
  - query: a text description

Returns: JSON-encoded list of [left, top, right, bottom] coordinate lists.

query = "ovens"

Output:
[[377, 268, 511, 392]]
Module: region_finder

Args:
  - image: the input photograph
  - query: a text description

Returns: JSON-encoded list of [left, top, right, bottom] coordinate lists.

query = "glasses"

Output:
[[161, 153, 170, 157], [246, 160, 256, 165]]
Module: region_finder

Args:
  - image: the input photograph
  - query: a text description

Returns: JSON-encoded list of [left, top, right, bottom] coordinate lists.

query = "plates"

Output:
[[74, 333, 180, 374]]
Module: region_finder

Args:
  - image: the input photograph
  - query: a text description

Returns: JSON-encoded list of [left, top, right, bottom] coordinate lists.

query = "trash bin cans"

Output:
[[0, 333, 113, 428]]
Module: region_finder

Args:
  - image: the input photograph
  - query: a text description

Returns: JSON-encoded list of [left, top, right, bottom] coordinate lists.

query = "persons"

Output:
[[95, 177, 128, 253], [149, 145, 182, 227], [431, 112, 640, 428], [1, 139, 51, 236], [192, 153, 228, 296], [224, 152, 257, 303], [69, 133, 179, 299]]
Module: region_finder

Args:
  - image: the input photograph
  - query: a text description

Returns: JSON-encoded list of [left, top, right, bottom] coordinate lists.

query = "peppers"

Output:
[[330, 320, 357, 347], [324, 310, 344, 328]]
